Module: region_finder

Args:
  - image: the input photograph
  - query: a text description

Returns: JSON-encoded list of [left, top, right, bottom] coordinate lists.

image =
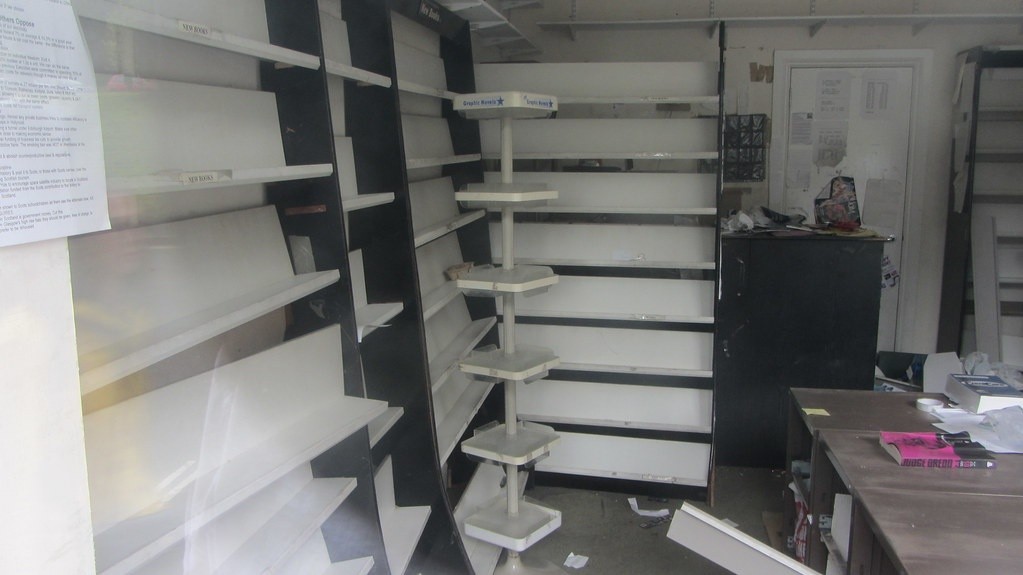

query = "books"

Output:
[[945, 374, 1023, 415], [879, 430, 996, 469]]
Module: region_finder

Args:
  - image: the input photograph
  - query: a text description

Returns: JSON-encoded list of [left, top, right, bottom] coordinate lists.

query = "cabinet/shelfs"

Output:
[[785, 385, 1023, 575], [937, 45, 1023, 372], [0, 0, 728, 575], [719, 234, 892, 467]]
